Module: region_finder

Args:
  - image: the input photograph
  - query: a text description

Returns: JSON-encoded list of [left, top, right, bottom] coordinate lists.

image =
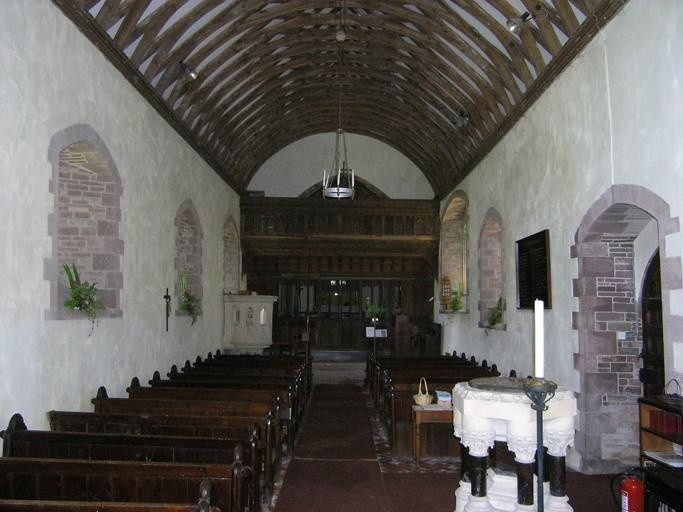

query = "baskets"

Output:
[[412, 376, 434, 406]]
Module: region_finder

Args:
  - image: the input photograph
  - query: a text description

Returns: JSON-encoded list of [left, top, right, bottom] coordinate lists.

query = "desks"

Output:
[[451, 381, 578, 512]]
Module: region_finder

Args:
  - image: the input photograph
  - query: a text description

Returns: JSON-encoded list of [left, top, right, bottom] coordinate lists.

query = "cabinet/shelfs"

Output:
[[636, 398, 683, 512]]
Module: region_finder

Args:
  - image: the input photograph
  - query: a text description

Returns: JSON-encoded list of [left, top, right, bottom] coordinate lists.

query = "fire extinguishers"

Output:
[[610, 466, 645, 512]]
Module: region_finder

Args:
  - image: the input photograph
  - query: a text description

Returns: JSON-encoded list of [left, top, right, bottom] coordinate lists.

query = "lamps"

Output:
[[322, 31, 356, 200], [507, 3, 549, 34], [180, 61, 197, 82]]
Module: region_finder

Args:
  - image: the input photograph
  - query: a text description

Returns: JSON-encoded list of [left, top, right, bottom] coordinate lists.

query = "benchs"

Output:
[[367, 352, 515, 467]]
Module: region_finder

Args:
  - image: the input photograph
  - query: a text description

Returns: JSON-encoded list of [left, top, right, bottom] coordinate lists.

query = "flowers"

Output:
[[64, 262, 105, 336], [179, 289, 202, 326]]
